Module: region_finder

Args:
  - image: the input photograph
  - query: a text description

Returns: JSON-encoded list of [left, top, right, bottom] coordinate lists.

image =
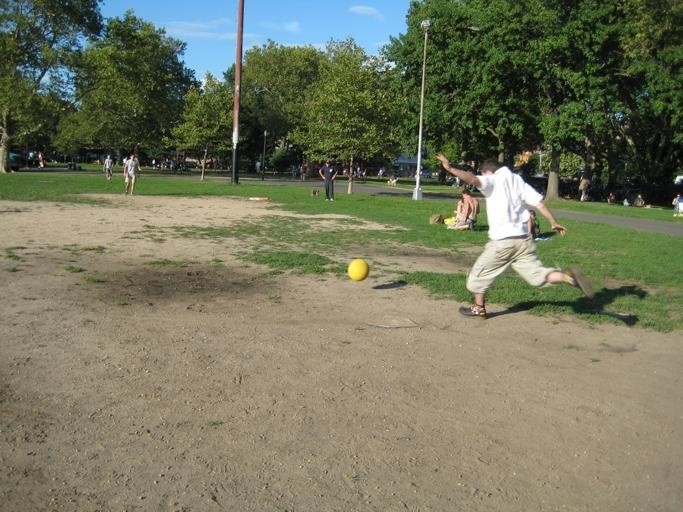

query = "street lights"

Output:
[[411, 20, 431, 200]]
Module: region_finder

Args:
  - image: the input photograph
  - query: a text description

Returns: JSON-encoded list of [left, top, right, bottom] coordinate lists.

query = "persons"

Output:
[[436, 168, 480, 230], [339, 160, 368, 183], [377, 165, 417, 188], [238, 158, 262, 176], [435, 150, 595, 324], [141, 156, 197, 171], [318, 160, 339, 201], [527, 210, 542, 239], [672, 193, 683, 217], [103, 154, 113, 184], [122, 153, 142, 196], [287, 164, 320, 183], [599, 190, 646, 209], [28, 150, 47, 171]]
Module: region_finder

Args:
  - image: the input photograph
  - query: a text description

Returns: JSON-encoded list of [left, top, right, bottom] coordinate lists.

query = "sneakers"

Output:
[[459, 303, 487, 320], [564, 264, 594, 301]]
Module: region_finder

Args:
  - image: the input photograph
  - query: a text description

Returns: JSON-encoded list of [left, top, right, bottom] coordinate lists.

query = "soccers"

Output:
[[348, 258, 368, 281]]
